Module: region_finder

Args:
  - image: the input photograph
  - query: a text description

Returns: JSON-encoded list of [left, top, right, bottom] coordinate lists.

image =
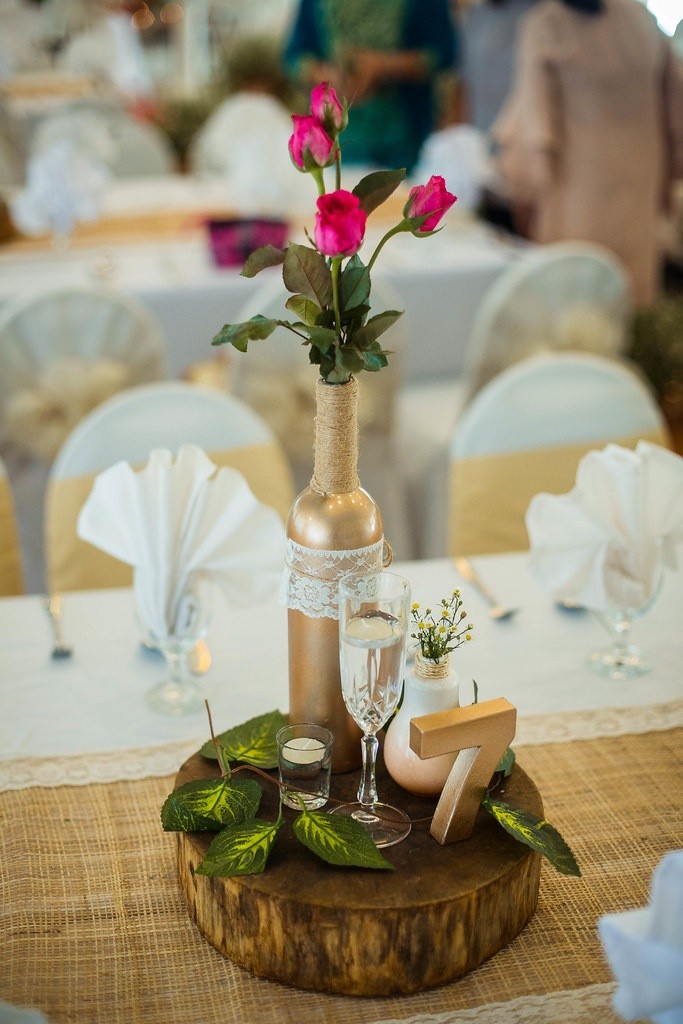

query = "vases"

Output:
[[383, 674, 466, 792], [284, 377, 385, 777]]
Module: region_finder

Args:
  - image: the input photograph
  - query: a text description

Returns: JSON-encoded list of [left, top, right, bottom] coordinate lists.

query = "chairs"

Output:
[[443, 347, 674, 554], [46, 381, 294, 595], [393, 235, 640, 563], [188, 100, 308, 185], [1, 281, 167, 597], [34, 112, 179, 195]]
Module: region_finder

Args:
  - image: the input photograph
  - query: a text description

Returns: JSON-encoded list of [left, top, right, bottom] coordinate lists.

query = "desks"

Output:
[[0, 556, 683, 1024], [0, 207, 541, 404]]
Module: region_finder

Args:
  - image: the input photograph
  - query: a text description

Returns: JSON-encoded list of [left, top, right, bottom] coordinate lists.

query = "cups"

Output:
[[276, 723, 335, 810]]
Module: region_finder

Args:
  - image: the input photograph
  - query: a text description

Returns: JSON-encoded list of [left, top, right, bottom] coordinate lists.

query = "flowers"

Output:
[[209, 78, 459, 383], [408, 590, 477, 675]]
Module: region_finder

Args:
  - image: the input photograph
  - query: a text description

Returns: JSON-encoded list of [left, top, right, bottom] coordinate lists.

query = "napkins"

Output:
[[75, 440, 290, 625], [596, 852, 683, 1024], [522, 438, 683, 619]]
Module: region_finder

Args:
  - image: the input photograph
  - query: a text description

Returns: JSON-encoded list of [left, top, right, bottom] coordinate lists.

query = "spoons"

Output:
[[454, 556, 518, 622]]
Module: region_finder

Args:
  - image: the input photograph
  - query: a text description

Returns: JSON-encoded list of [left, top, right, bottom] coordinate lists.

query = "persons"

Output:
[[278, 1, 683, 316]]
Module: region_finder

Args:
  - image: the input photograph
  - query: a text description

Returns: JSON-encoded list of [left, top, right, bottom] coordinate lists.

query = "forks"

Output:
[[47, 597, 73, 659]]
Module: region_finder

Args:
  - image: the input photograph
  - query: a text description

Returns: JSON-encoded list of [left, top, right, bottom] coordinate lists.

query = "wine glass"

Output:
[[585, 541, 664, 682], [326, 571, 412, 849], [134, 567, 220, 714]]
